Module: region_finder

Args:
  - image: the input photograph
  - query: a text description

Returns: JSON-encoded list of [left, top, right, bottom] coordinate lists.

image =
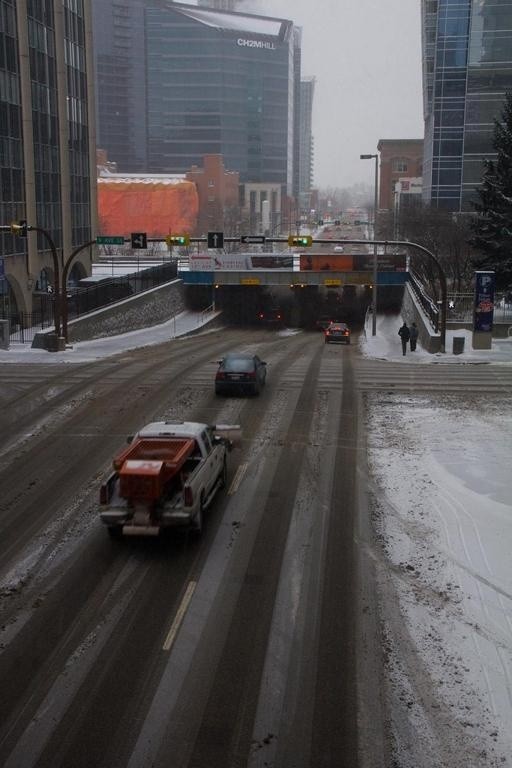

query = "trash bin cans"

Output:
[[47, 334, 58, 352], [453, 337, 465, 354]]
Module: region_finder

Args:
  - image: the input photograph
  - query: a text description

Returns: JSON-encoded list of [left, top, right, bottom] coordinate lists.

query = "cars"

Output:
[[243, 284, 374, 346], [316, 215, 363, 253], [212, 351, 269, 398]]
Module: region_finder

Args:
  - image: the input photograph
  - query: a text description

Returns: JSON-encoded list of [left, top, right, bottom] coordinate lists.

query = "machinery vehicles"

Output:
[[91, 419, 243, 542]]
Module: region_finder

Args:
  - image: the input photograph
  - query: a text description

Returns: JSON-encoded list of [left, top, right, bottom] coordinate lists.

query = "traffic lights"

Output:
[[447, 299, 457, 310], [286, 235, 313, 247], [10, 221, 24, 235], [165, 233, 190, 246], [27, 225, 33, 232], [46, 284, 54, 294]]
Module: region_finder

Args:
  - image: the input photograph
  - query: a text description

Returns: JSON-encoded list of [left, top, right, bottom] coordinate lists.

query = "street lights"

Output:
[[358, 153, 379, 338]]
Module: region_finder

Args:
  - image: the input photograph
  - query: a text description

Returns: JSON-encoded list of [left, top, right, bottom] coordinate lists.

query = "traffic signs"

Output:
[[20, 220, 28, 237], [96, 236, 125, 245]]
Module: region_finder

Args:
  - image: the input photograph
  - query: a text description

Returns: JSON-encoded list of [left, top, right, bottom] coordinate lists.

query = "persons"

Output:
[[398, 321, 419, 356]]
